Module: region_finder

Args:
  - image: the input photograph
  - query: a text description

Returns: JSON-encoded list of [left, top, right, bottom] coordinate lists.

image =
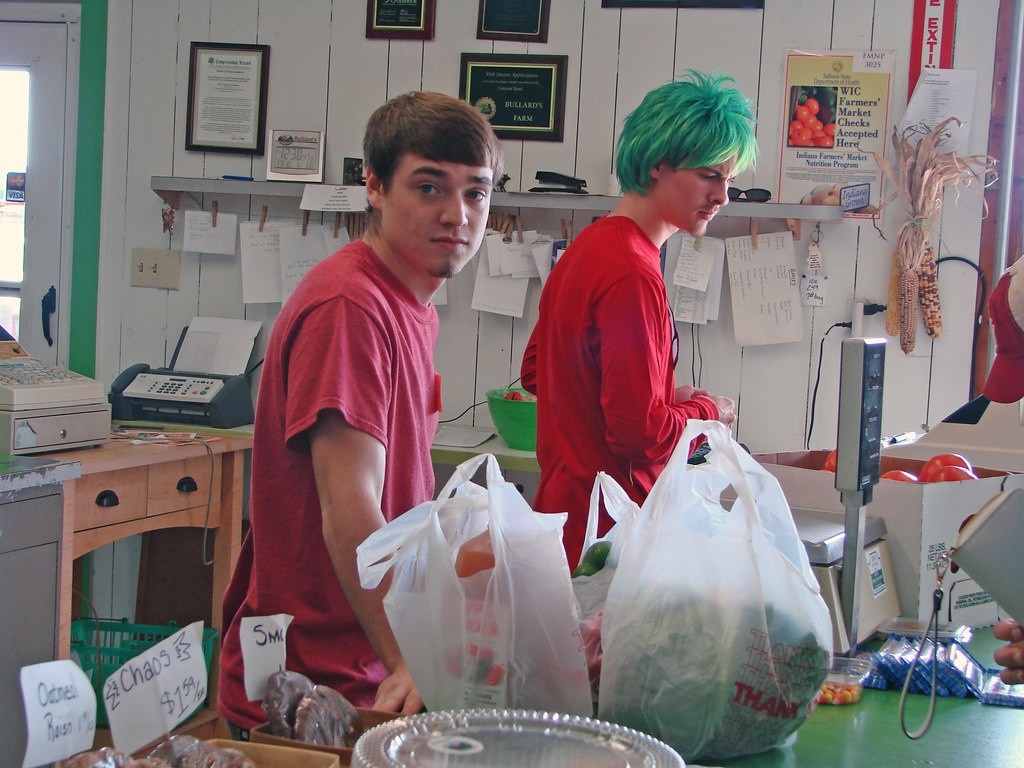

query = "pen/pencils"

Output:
[[222, 175, 253, 181]]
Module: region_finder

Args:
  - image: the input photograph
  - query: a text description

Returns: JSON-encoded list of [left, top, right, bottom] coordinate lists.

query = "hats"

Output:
[[983, 255, 1024, 403]]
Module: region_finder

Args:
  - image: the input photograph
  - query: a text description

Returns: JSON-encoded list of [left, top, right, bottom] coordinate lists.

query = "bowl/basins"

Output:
[[485, 388, 536, 453]]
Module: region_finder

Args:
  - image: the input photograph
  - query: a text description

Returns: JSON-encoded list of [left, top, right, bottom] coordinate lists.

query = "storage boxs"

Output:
[[70, 614, 406, 768], [752, 451, 1024, 629]]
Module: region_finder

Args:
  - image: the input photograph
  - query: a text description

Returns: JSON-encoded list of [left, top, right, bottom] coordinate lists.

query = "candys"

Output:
[[820, 684, 860, 704]]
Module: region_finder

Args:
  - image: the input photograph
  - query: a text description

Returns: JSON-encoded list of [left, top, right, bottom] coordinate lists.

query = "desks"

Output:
[[112, 422, 542, 473], [0, 456, 82, 768], [689, 631, 1024, 767], [34, 435, 253, 713]]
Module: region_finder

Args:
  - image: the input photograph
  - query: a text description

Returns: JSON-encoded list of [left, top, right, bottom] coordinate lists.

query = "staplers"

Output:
[[528, 171, 589, 194]]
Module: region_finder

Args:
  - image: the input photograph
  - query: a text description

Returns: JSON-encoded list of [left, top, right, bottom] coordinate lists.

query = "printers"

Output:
[[108, 326, 255, 430]]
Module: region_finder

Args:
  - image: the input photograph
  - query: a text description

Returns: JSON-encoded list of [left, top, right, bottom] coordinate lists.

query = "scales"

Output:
[[718, 335, 900, 658]]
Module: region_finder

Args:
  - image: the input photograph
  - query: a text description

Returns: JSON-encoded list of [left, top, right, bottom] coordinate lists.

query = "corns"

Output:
[[886, 248, 943, 354]]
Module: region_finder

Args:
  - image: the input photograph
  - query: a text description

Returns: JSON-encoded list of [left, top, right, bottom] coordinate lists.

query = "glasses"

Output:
[[727, 187, 772, 202]]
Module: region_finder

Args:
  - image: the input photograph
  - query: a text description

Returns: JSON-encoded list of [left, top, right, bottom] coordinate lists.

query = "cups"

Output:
[[343, 157, 363, 186], [607, 173, 624, 197]]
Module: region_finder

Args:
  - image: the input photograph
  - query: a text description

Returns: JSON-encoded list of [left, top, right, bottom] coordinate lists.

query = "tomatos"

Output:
[[789, 99, 836, 147], [880, 454, 979, 483], [819, 450, 838, 473]]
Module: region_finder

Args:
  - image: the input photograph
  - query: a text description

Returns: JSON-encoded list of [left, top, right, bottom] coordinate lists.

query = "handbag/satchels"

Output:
[[354, 417, 834, 764]]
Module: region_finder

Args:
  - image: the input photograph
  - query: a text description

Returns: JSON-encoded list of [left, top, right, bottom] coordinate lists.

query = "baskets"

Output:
[[67, 615, 219, 731]]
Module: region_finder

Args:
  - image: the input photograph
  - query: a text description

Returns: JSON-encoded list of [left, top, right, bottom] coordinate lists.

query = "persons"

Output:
[[520, 64, 757, 574], [214, 88, 502, 742]]
